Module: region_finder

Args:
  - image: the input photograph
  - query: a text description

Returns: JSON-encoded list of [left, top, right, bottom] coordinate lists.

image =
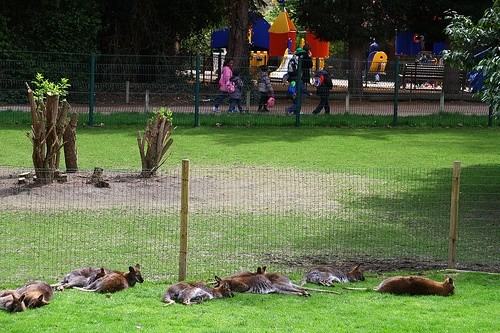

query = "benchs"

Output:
[[398, 63, 467, 90]]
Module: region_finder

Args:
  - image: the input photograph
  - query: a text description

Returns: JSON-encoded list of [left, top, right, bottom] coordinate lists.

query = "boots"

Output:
[[257, 105, 263, 112], [263, 104, 269, 112]]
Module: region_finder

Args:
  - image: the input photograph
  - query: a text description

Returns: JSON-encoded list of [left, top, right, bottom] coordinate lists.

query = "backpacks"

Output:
[[287, 81, 297, 100], [219, 69, 226, 86], [288, 54, 300, 77], [228, 80, 238, 94], [315, 74, 328, 87]]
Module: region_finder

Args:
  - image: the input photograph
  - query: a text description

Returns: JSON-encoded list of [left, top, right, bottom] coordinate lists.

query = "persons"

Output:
[[312, 68, 333, 115], [212, 60, 245, 114], [257, 44, 313, 115]]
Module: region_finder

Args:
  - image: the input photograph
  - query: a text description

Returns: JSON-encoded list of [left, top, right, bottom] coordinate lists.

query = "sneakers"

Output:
[[227, 110, 233, 114], [213, 107, 221, 113], [285, 108, 289, 116], [232, 109, 238, 113], [240, 110, 246, 113]]
